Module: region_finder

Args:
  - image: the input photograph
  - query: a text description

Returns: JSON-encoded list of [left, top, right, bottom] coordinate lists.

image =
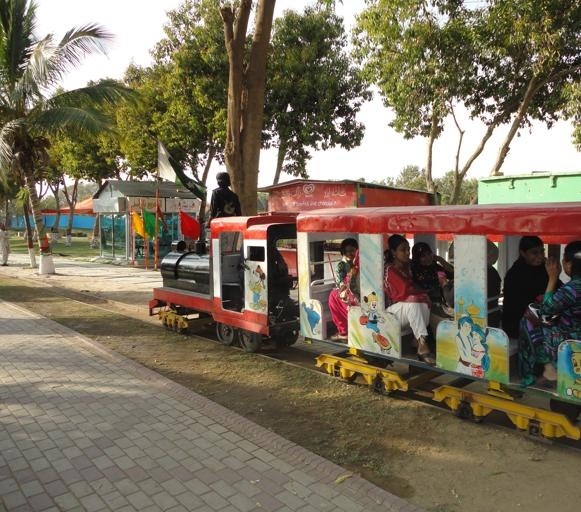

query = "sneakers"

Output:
[[330, 334, 348, 344]]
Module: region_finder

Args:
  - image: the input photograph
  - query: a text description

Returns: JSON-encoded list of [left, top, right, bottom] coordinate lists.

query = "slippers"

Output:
[[416, 349, 437, 366], [534, 373, 558, 390]]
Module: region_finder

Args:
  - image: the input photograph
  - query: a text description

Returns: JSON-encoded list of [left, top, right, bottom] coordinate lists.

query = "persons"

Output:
[[455, 317, 486, 376], [516, 240, 580, 388], [204, 171, 243, 230], [328, 234, 503, 368], [500, 234, 566, 378], [460, 324, 491, 379], [0, 223, 11, 266]]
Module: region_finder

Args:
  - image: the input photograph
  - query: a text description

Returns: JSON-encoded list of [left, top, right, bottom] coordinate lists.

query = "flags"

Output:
[[158, 205, 169, 230], [143, 208, 163, 240], [157, 140, 177, 184], [131, 207, 151, 241], [180, 210, 202, 242]]
